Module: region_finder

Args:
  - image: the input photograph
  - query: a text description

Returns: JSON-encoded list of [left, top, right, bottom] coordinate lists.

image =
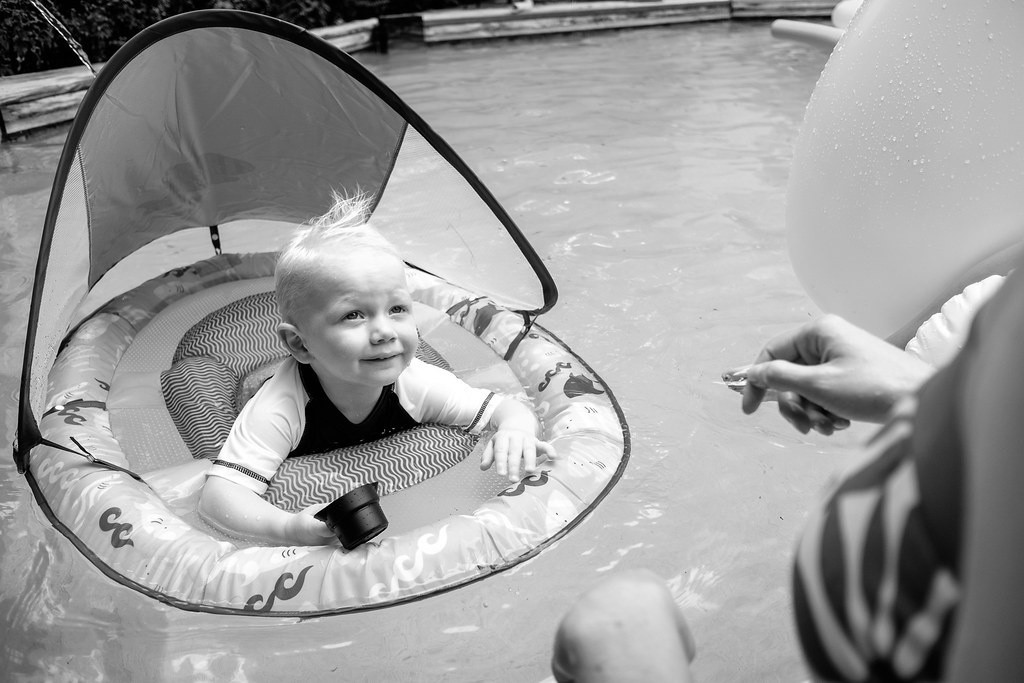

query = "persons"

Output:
[[552, 262, 1024, 683], [196, 190, 556, 548]]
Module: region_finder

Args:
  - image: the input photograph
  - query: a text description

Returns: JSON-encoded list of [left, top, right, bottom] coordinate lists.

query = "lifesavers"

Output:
[[785, 0, 1024, 352], [769, 17, 845, 49]]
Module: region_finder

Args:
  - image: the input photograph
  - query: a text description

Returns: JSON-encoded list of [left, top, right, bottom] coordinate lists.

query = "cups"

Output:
[[313, 482, 389, 550]]
